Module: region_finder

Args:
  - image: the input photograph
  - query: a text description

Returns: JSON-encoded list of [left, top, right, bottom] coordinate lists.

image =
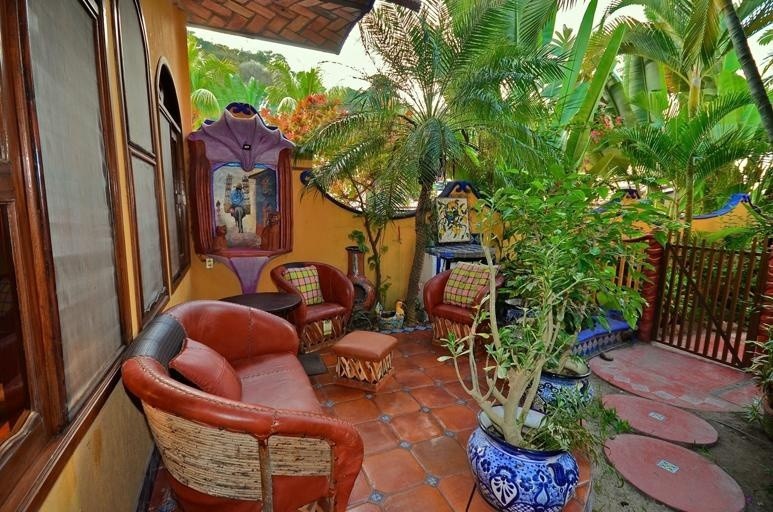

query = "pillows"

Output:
[[282, 265, 325, 304], [167, 337, 242, 403], [443, 262, 501, 307]]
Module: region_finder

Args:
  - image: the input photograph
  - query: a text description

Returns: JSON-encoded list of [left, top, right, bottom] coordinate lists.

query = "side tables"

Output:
[[424, 244, 495, 275], [218, 292, 302, 325]]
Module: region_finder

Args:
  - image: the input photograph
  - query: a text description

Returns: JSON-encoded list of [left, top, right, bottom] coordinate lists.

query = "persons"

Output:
[[230, 183, 245, 233]]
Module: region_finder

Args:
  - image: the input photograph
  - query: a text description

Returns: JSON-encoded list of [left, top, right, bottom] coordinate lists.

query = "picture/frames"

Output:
[[435, 197, 471, 243]]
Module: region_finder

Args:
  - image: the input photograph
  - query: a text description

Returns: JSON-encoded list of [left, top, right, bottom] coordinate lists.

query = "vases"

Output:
[[345, 245, 375, 315]]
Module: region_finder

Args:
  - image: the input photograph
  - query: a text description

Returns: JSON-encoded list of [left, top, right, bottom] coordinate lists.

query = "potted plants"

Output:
[[435, 166, 620, 512], [512, 177, 690, 421]]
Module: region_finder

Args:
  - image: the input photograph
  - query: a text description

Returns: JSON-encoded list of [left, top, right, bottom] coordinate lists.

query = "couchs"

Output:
[[120, 299, 364, 512]]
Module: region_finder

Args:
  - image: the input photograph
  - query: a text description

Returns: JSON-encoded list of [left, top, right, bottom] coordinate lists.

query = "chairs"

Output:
[[270, 262, 354, 354], [423, 269, 507, 359]]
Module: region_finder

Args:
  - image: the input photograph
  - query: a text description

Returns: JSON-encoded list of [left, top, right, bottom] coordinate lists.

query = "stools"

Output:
[[333, 329, 399, 392]]
